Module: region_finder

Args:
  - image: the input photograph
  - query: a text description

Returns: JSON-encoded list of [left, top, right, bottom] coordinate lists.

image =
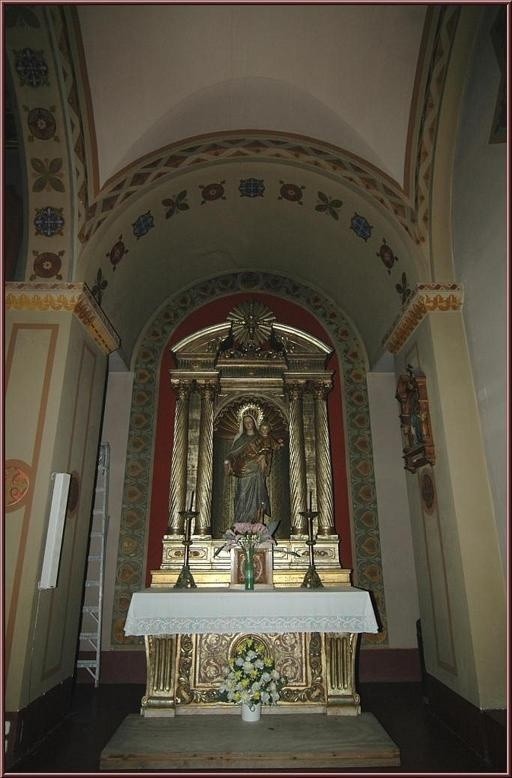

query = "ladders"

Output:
[[76, 441, 111, 688]]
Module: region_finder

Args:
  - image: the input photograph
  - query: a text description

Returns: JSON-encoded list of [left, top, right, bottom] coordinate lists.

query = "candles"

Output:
[[188, 489, 194, 512], [306, 490, 311, 510]]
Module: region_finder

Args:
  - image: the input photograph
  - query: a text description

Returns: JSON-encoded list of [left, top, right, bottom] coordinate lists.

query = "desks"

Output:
[[121, 586, 380, 637]]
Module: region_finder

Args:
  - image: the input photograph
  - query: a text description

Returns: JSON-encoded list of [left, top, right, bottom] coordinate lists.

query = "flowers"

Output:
[[215, 637, 288, 712], [213, 522, 302, 558]]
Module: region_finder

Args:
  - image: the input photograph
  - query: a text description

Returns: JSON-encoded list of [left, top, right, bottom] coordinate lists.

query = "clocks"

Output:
[[394, 363, 436, 515]]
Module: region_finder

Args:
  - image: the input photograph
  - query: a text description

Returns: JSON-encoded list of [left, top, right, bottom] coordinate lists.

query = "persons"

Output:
[[224, 414, 271, 526], [252, 423, 280, 477]]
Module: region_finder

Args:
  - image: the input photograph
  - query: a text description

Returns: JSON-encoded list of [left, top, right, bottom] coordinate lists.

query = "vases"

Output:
[[241, 703, 261, 722], [244, 551, 255, 590]]
